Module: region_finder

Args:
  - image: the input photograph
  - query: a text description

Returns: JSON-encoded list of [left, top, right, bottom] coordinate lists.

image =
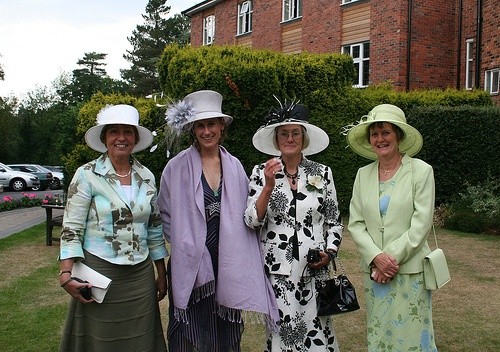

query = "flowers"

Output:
[[340, 111, 376, 149], [145, 91, 199, 159], [306, 174, 324, 193], [95, 103, 114, 125], [257, 93, 310, 127]]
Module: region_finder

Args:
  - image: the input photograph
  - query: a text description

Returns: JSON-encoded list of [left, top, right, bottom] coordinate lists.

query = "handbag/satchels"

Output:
[[315, 250, 360, 316], [423, 224, 451, 290], [70, 262, 112, 304]]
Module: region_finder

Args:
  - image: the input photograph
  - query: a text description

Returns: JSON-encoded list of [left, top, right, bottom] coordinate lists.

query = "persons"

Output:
[[346, 104, 438, 352], [57, 104, 170, 351], [245, 104, 344, 352], [156, 90, 279, 352]]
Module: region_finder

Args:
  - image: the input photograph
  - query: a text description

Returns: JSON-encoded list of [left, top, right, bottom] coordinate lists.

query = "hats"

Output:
[[146, 90, 233, 158], [341, 104, 423, 161], [85, 103, 154, 153], [252, 94, 330, 157]]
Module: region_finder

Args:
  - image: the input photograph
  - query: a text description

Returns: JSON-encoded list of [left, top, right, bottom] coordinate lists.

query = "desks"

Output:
[[41, 204, 66, 246]]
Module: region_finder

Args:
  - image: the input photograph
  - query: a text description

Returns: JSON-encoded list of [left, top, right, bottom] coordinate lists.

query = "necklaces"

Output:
[[380, 155, 402, 177], [116, 166, 131, 178], [202, 164, 220, 197], [285, 169, 298, 185]]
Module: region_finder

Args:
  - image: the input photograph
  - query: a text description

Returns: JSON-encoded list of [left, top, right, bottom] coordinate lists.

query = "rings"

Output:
[[382, 281, 385, 283]]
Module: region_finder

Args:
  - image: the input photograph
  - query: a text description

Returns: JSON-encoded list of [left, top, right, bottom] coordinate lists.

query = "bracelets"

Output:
[[59, 270, 72, 276]]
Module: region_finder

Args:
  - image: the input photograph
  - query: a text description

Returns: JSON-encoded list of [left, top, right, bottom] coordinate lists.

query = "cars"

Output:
[[0, 163, 40, 192], [8, 166, 53, 191], [5, 165, 65, 190]]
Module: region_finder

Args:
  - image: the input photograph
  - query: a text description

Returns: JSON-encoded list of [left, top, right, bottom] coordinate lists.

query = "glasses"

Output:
[[277, 131, 304, 139]]
